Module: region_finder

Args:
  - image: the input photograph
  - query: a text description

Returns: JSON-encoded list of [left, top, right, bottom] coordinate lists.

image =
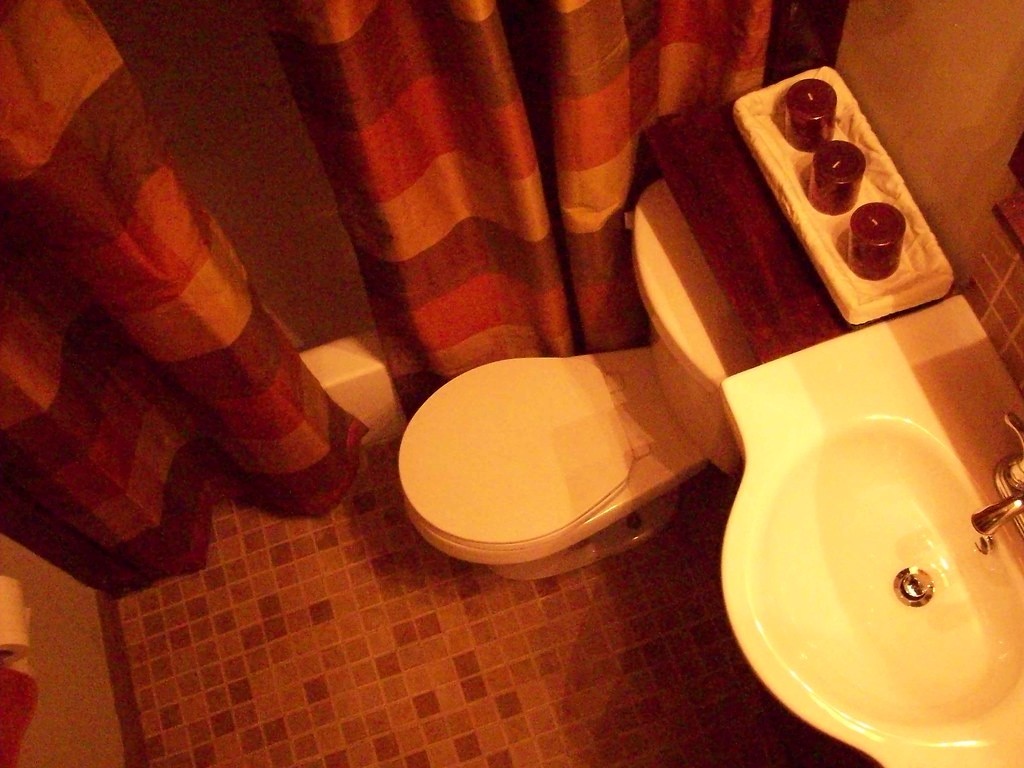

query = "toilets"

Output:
[[395, 175, 741, 583]]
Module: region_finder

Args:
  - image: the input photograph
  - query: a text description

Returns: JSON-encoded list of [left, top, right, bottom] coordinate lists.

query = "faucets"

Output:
[[969, 493, 1024, 538]]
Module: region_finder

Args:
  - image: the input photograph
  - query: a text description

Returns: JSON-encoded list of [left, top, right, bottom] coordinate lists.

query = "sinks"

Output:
[[718, 293, 1024, 768]]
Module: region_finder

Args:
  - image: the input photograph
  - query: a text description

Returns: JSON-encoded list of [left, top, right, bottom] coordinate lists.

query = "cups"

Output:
[[807, 140, 866, 216], [784, 79, 838, 152], [848, 202, 907, 280]]
